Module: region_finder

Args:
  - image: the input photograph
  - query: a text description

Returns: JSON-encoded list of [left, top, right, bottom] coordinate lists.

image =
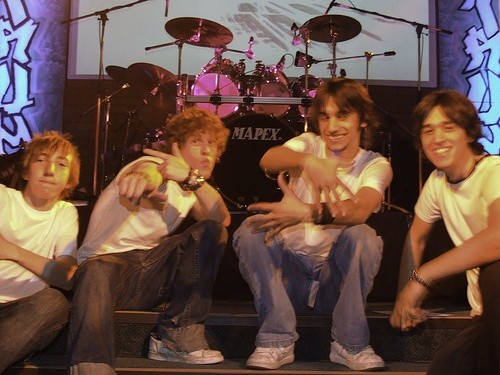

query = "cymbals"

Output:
[[126, 62, 178, 112], [299, 14, 362, 43], [105, 65, 127, 85], [165, 17, 234, 48]]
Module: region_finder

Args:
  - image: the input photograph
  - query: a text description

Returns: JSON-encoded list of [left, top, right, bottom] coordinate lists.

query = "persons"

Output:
[[0, 130, 80, 375], [390, 90, 500, 375], [232, 78, 393, 370], [68, 107, 232, 375]]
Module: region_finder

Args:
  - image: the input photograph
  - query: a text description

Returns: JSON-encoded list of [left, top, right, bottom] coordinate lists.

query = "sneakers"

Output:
[[247, 342, 294, 369], [148, 333, 224, 365], [329, 339, 385, 371]]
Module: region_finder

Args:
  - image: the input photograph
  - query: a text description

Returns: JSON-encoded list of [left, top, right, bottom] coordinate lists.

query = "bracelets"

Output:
[[409, 270, 432, 290], [316, 203, 335, 225], [180, 167, 205, 191]]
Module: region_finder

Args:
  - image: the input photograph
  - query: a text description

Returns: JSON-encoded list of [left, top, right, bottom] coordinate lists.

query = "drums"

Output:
[[291, 74, 325, 118], [190, 59, 248, 120], [209, 111, 300, 210], [245, 63, 293, 118]]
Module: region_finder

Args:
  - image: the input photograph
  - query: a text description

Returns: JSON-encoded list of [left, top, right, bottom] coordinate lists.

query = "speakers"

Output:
[[364, 201, 411, 304]]
